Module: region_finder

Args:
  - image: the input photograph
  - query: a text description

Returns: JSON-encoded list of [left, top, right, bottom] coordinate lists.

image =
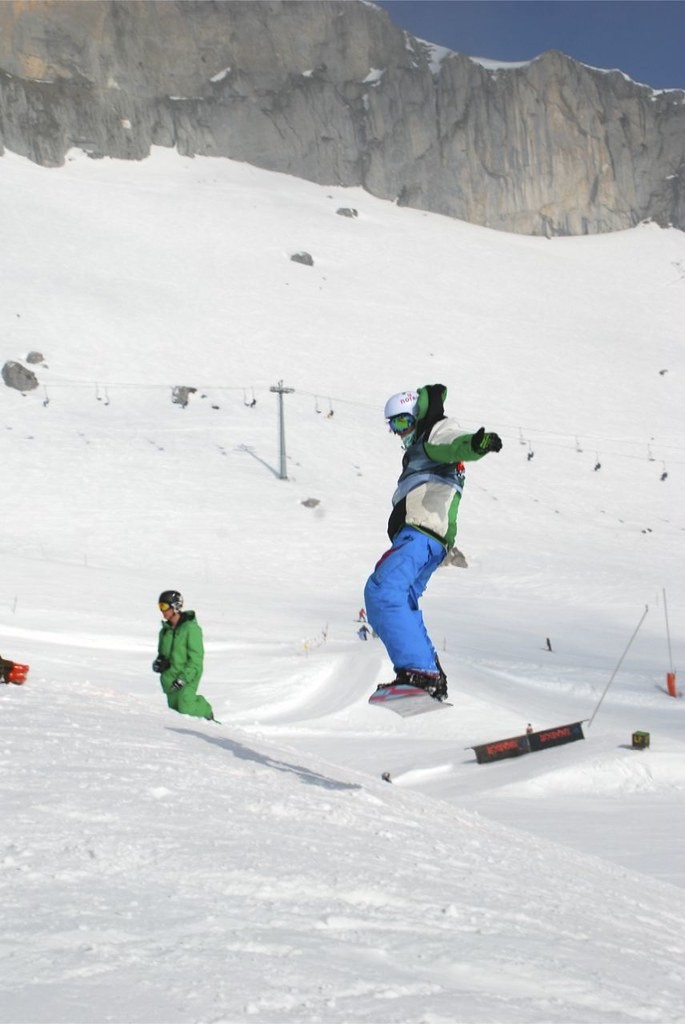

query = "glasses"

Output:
[[389, 415, 416, 434], [159, 603, 171, 610]]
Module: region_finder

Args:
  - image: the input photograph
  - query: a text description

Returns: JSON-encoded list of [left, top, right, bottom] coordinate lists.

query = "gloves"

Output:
[[472, 427, 502, 452], [171, 679, 185, 690]]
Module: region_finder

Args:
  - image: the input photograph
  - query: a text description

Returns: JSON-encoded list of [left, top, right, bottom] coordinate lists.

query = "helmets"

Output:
[[159, 591, 183, 614], [385, 391, 422, 449]]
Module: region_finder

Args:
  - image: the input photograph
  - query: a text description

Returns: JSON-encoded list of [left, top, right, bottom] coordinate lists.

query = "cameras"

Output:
[[153, 656, 170, 673]]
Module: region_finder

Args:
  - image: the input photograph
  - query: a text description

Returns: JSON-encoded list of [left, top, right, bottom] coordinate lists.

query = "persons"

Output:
[[364, 384, 502, 700], [360, 625, 369, 640], [359, 608, 366, 621], [153, 591, 214, 721]]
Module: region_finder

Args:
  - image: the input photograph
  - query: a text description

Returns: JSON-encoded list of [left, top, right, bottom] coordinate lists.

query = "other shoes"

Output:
[[377, 670, 445, 699]]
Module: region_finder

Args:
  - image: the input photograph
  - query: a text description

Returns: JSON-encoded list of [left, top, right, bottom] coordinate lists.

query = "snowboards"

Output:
[[367, 683, 454, 718]]
